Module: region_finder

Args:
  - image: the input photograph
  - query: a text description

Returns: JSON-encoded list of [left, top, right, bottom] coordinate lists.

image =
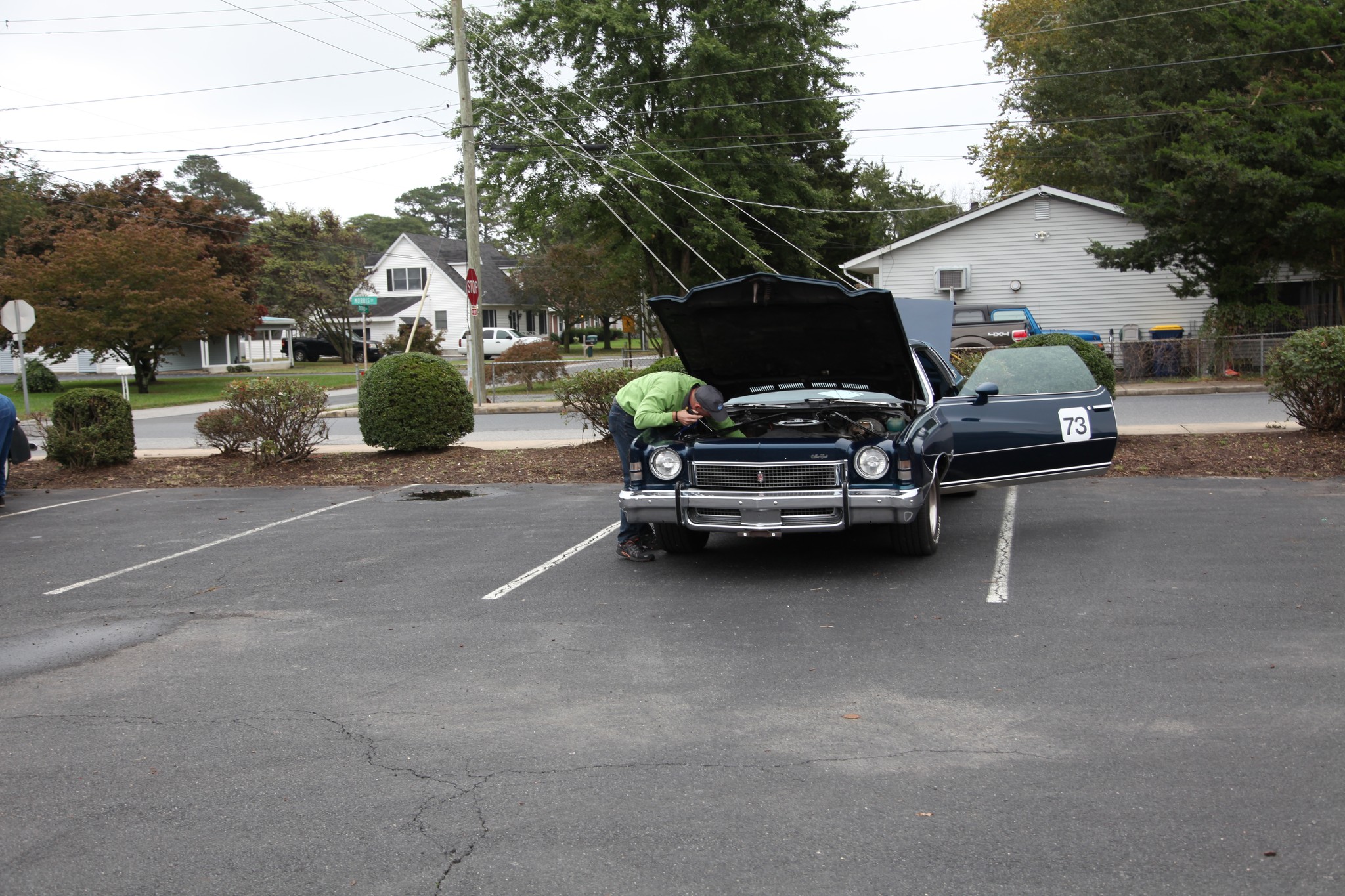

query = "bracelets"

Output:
[[675, 411, 678, 422]]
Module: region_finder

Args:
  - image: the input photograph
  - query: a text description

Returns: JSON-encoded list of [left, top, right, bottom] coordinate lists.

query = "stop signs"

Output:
[[466, 268, 479, 306]]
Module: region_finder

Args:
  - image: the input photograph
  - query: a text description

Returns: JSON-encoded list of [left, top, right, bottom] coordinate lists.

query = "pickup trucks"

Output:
[[950, 319, 1032, 361], [280, 329, 386, 364]]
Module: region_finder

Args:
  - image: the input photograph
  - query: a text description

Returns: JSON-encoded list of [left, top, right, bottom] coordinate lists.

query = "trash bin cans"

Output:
[[1119, 323, 1150, 378], [1149, 324, 1185, 377]]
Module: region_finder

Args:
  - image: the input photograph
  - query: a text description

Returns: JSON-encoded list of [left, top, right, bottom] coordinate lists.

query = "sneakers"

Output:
[[616, 538, 655, 562], [638, 525, 659, 550]]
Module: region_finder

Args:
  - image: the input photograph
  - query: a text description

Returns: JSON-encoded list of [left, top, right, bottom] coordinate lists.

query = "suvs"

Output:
[[953, 304, 1105, 351], [458, 327, 545, 360]]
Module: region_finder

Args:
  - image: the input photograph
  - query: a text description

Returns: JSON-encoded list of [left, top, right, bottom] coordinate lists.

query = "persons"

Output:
[[0, 394, 17, 509], [608, 371, 748, 561]]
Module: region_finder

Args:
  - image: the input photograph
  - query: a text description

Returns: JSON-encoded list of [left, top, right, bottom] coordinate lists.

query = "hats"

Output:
[[695, 385, 728, 422]]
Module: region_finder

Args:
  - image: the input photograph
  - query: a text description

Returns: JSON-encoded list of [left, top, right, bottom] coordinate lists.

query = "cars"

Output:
[[617, 270, 1119, 560]]
